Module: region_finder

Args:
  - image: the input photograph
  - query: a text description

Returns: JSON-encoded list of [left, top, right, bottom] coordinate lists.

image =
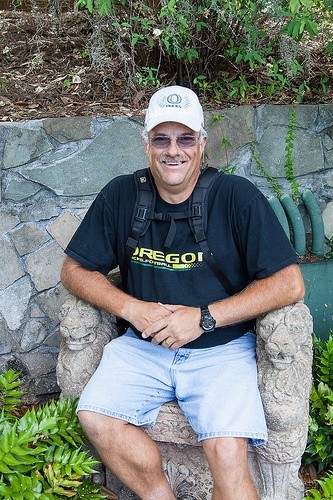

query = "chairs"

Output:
[[55, 271, 313, 498]]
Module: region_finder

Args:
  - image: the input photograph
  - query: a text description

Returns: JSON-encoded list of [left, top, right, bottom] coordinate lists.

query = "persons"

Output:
[[60, 83, 306, 499]]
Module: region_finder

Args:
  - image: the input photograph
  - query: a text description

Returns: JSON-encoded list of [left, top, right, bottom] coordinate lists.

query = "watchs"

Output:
[[198, 304, 217, 334]]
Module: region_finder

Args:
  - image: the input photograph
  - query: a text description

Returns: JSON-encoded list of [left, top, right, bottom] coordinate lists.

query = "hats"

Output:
[[145, 85, 205, 132]]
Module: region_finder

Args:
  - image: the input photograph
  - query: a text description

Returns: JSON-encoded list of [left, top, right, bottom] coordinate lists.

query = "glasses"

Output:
[[148, 135, 200, 149]]
